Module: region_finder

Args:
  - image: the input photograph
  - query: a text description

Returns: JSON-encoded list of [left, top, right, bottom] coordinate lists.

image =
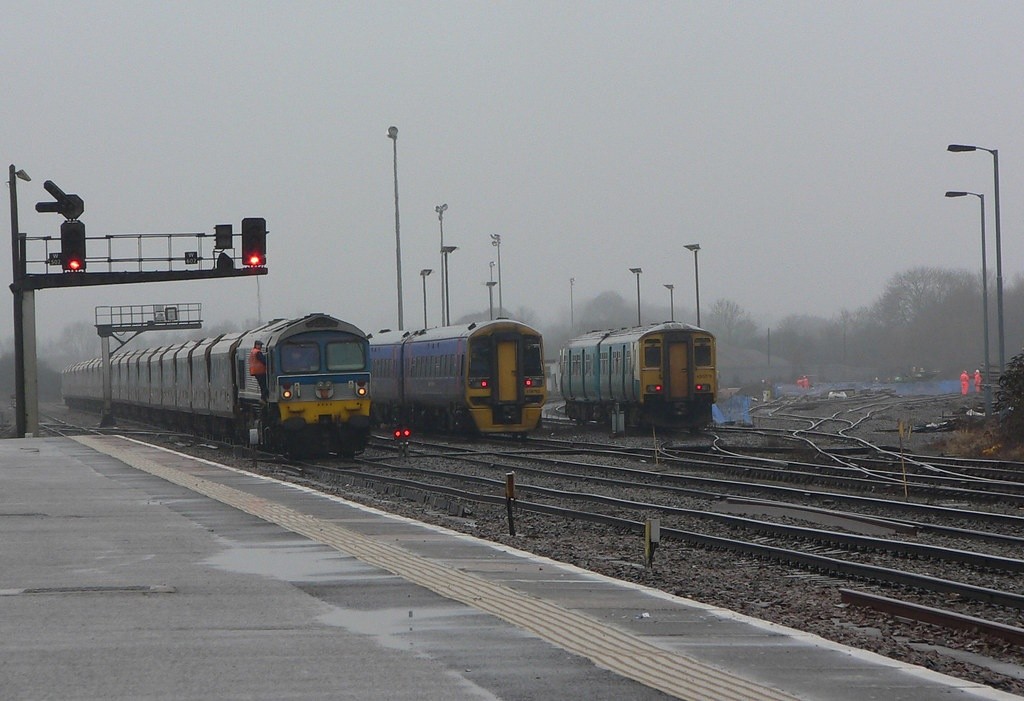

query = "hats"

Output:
[[255, 341, 264, 345]]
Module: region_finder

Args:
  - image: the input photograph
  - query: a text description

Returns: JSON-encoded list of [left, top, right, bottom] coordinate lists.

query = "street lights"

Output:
[[948, 145, 1007, 428], [485, 261, 498, 319], [569, 277, 577, 330], [440, 246, 458, 326], [684, 243, 701, 327], [945, 191, 992, 418], [387, 126, 404, 331], [435, 203, 448, 327], [419, 268, 433, 328], [490, 232, 505, 317], [664, 284, 675, 321], [629, 267, 643, 324]]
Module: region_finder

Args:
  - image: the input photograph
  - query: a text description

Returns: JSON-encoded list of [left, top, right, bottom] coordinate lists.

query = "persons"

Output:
[[961, 371, 969, 395], [250, 340, 271, 405], [975, 370, 983, 392], [290, 348, 310, 372], [470, 346, 490, 377], [797, 376, 811, 389]]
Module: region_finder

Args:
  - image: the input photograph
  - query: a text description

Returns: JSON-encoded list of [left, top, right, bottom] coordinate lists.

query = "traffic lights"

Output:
[[241, 217, 267, 265], [61, 220, 86, 270], [403, 426, 410, 440], [394, 426, 403, 440]]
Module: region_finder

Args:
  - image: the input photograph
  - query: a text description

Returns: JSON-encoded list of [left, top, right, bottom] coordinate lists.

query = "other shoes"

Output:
[[261, 397, 268, 404]]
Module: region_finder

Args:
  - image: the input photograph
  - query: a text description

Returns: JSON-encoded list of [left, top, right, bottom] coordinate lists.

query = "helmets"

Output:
[[976, 370, 979, 372], [964, 368, 968, 372]]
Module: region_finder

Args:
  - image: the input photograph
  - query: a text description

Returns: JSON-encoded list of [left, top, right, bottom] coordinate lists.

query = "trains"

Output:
[[559, 320, 718, 430], [368, 319, 547, 439], [61, 312, 373, 461]]
